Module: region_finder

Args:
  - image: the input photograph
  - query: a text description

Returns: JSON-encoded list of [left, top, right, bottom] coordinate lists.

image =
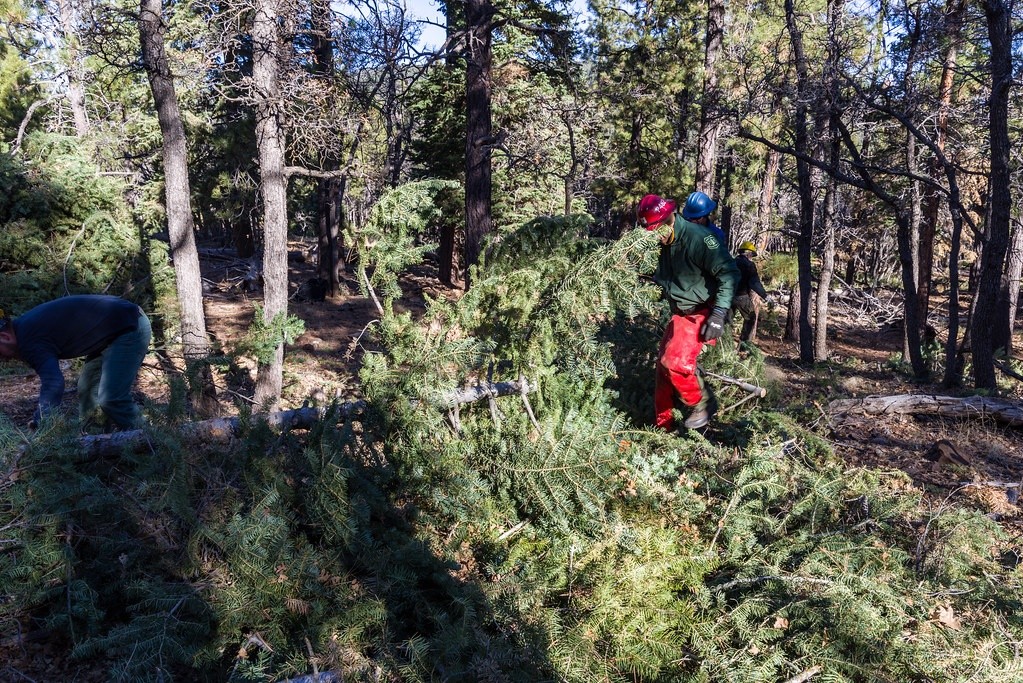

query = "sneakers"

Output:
[[685, 406, 717, 428], [738, 351, 750, 359]]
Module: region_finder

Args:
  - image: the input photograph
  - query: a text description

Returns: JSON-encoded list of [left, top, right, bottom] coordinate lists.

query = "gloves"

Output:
[[768, 298, 775, 310], [701, 307, 727, 341]]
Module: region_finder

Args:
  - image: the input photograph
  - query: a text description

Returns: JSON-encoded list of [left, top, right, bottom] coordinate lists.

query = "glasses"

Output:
[[690, 215, 706, 220]]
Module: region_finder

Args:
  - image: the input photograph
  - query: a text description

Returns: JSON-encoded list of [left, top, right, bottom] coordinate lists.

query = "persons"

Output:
[[681, 192, 727, 350], [0, 294, 152, 476], [636, 193, 743, 451], [734, 241, 773, 362]]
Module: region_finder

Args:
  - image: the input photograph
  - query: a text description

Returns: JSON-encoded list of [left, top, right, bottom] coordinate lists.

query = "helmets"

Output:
[[638, 194, 676, 225], [682, 192, 717, 219], [737, 241, 758, 257]]
